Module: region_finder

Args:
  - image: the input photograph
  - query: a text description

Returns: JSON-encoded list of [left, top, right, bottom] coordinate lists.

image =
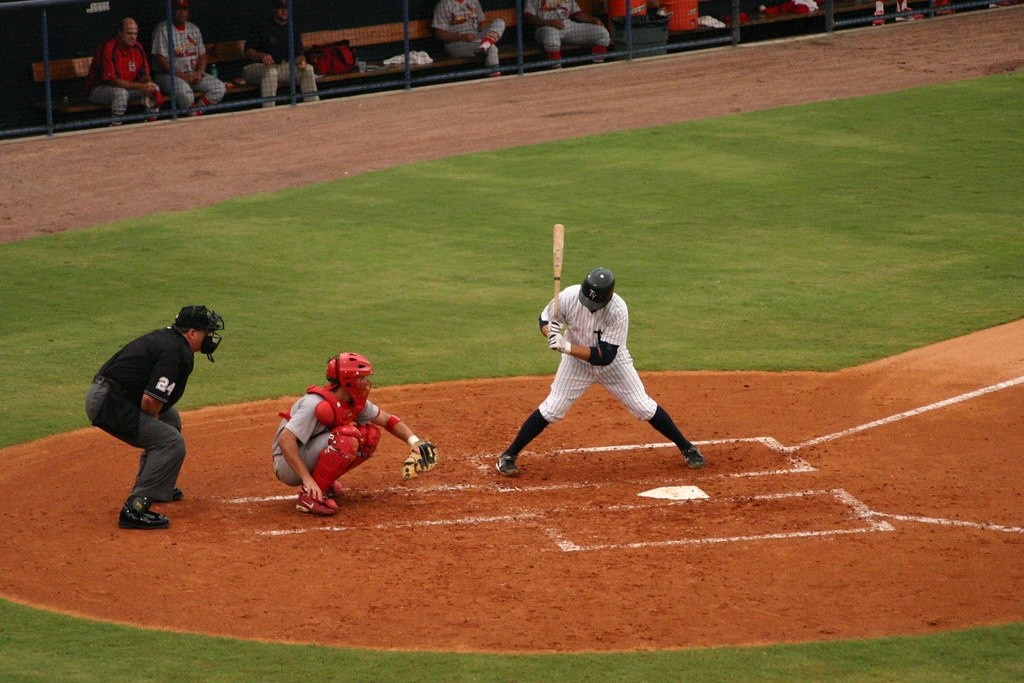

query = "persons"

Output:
[[524, 0, 610, 70], [150, 0, 225, 118], [84, 17, 159, 127], [242, 0, 320, 108], [86, 305, 224, 528], [431, 0, 506, 78], [272, 352, 426, 514], [871, 0, 954, 26], [496, 268, 705, 475]]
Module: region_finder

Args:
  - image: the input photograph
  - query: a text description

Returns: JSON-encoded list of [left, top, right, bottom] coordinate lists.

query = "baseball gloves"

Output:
[[402, 440, 439, 481]]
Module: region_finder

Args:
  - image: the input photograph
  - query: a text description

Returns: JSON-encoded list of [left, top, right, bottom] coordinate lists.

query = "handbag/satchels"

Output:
[[311, 44, 355, 76]]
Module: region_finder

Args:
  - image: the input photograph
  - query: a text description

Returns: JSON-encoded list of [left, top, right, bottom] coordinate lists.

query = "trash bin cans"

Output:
[[610, 12, 673, 61]]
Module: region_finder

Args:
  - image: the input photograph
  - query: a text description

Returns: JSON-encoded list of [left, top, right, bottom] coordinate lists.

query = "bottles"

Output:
[[211, 63, 217, 78]]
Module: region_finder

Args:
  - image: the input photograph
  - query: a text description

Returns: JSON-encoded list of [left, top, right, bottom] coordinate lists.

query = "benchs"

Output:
[[31, 0, 914, 117]]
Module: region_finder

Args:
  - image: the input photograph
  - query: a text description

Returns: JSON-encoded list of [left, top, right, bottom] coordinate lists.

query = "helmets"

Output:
[[175, 305, 211, 330], [326, 353, 373, 411], [579, 268, 615, 311]]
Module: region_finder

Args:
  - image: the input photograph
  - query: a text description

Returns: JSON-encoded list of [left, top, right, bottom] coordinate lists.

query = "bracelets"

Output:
[[407, 435, 420, 446]]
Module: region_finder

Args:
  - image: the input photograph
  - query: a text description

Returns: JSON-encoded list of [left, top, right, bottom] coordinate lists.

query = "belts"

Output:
[[92, 377, 112, 387]]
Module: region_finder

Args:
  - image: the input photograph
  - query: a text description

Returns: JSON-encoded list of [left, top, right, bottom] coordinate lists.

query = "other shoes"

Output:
[[474, 47, 489, 61]]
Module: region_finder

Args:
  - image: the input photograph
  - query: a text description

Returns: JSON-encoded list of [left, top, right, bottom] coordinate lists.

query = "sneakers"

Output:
[[153, 485, 183, 502], [682, 445, 705, 469], [895, 7, 925, 20], [302, 480, 345, 497], [296, 494, 338, 516], [118, 504, 170, 529], [495, 454, 519, 476], [872, 11, 885, 26]]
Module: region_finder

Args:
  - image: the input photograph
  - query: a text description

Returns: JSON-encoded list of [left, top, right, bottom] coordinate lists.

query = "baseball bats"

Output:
[[553, 223, 565, 321]]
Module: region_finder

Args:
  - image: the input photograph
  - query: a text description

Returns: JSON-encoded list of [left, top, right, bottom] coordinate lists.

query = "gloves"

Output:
[[547, 332, 573, 354], [548, 320, 563, 334]]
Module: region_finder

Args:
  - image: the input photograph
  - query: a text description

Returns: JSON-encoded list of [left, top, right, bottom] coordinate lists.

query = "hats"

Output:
[[140, 89, 164, 109], [171, 0, 190, 9], [270, 0, 289, 9]]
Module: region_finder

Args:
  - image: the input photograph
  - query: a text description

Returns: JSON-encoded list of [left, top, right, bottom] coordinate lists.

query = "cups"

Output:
[[358, 61, 366, 73]]
